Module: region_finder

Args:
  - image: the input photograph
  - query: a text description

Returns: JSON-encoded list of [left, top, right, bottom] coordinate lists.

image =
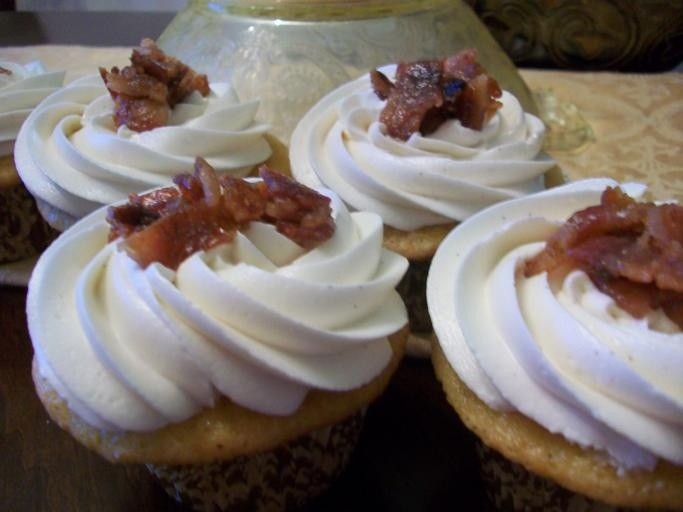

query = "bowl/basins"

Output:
[[155, 1, 595, 161]]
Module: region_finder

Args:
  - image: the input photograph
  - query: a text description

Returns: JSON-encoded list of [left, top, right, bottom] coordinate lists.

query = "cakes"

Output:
[[14, 38, 273, 233], [25, 155, 410, 512], [426, 177, 682, 510], [0, 60, 64, 262], [287, 48, 558, 261]]
